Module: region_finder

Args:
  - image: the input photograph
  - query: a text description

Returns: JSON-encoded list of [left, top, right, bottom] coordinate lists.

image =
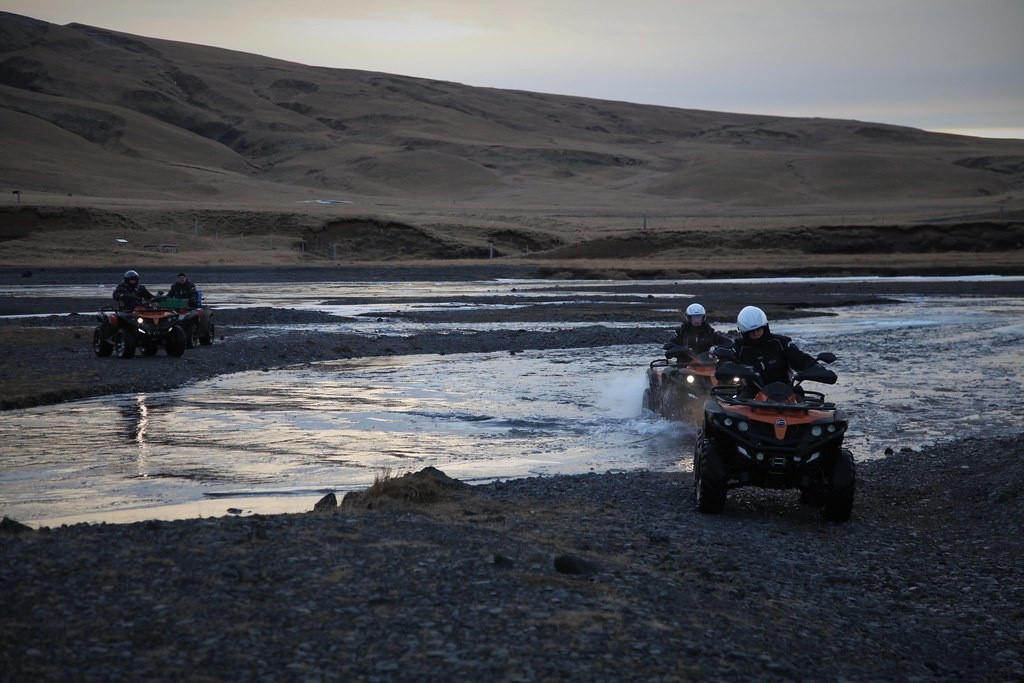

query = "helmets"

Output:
[[123, 270, 139, 288], [737, 305, 768, 333], [685, 303, 706, 322]]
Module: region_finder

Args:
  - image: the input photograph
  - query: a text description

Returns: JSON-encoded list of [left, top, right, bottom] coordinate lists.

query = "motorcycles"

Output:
[[94, 289, 215, 359], [693, 347, 857, 522], [642, 342, 740, 429]]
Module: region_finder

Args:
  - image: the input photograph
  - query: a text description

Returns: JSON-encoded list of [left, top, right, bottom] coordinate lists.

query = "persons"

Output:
[[112, 270, 154, 310], [715, 306, 835, 398], [668, 304, 733, 361], [166, 273, 197, 306]]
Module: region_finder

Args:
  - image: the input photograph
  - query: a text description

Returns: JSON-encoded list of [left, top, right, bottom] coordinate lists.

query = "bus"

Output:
[[140, 244, 181, 253]]
